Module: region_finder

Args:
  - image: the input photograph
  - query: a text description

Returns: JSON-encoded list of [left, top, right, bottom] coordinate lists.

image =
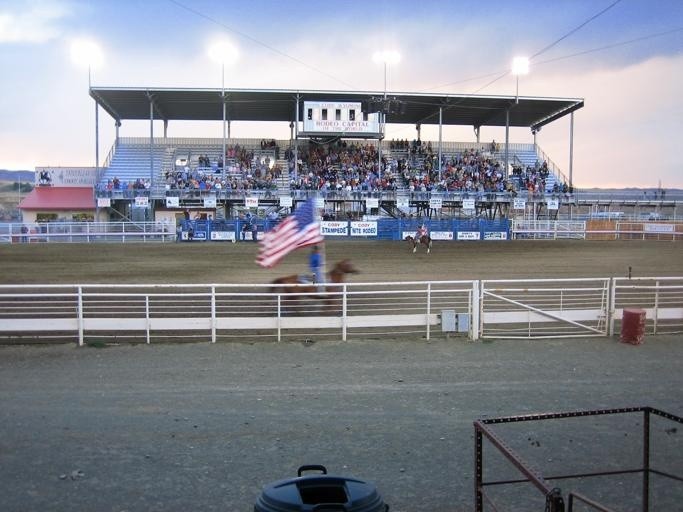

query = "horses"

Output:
[[406, 234, 433, 254], [267, 257, 360, 316]]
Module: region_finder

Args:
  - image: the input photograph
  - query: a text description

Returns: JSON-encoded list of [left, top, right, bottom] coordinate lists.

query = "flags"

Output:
[[255, 196, 324, 271]]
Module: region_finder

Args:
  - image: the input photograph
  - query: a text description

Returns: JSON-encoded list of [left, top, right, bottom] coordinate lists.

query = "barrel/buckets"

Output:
[[29, 230, 38, 242], [12, 232, 20, 243], [621, 307, 646, 346]]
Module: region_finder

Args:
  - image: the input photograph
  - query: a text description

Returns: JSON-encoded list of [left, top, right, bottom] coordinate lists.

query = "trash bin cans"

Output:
[[255, 465, 390, 512]]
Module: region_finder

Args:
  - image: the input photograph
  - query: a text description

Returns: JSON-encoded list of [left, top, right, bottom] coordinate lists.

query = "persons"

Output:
[[653, 191, 657, 200], [440, 213, 448, 220], [346, 210, 353, 218], [408, 213, 415, 219], [308, 244, 325, 297], [391, 138, 513, 198], [322, 213, 331, 221], [226, 139, 282, 196], [660, 188, 665, 200], [414, 225, 426, 244], [20, 222, 29, 244], [400, 213, 405, 218], [284, 136, 397, 198], [104, 177, 150, 198], [174, 208, 276, 243], [514, 159, 569, 197], [164, 154, 223, 198]]
